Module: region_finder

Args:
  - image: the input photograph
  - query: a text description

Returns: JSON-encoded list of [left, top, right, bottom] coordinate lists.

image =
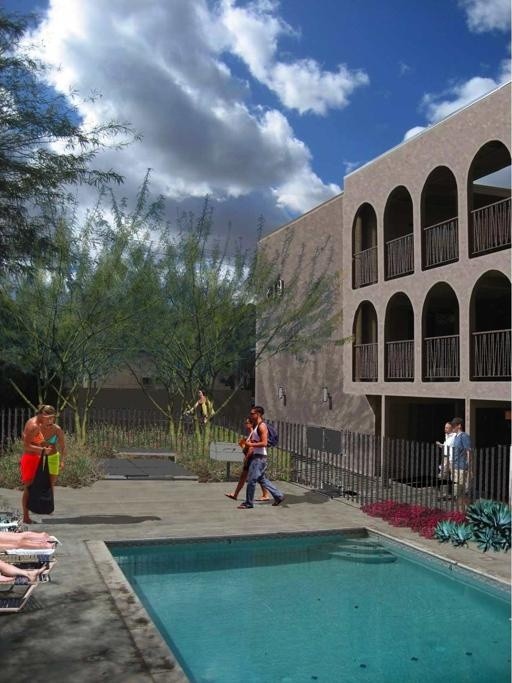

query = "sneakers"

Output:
[[224, 493, 237, 500], [272, 496, 287, 506], [256, 496, 269, 501], [238, 504, 253, 508]]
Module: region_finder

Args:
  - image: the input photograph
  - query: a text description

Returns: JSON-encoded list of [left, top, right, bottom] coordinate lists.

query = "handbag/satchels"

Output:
[[27, 472, 54, 514], [257, 421, 278, 446]]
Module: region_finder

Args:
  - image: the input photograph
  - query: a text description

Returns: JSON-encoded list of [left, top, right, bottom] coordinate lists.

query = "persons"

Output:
[[234, 405, 288, 510], [435, 422, 458, 503], [448, 417, 472, 505], [36, 421, 66, 513], [0, 560, 47, 583], [185, 388, 217, 425], [20, 402, 56, 525], [0, 530, 57, 549], [223, 417, 271, 502]]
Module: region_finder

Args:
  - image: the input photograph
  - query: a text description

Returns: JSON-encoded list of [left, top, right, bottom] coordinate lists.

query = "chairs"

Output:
[[0, 510, 58, 615]]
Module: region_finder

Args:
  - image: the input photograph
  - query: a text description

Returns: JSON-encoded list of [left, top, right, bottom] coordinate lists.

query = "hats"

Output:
[[448, 417, 464, 424]]
